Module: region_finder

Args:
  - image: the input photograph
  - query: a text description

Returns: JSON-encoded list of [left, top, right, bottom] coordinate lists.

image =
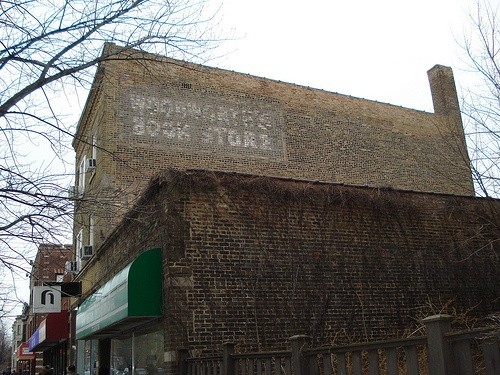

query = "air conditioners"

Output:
[[79, 245, 93, 258], [85, 158, 96, 171], [69, 186, 79, 199]]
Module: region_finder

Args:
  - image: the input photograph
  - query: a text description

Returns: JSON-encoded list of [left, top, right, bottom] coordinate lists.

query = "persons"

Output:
[[0, 364, 81, 375]]
